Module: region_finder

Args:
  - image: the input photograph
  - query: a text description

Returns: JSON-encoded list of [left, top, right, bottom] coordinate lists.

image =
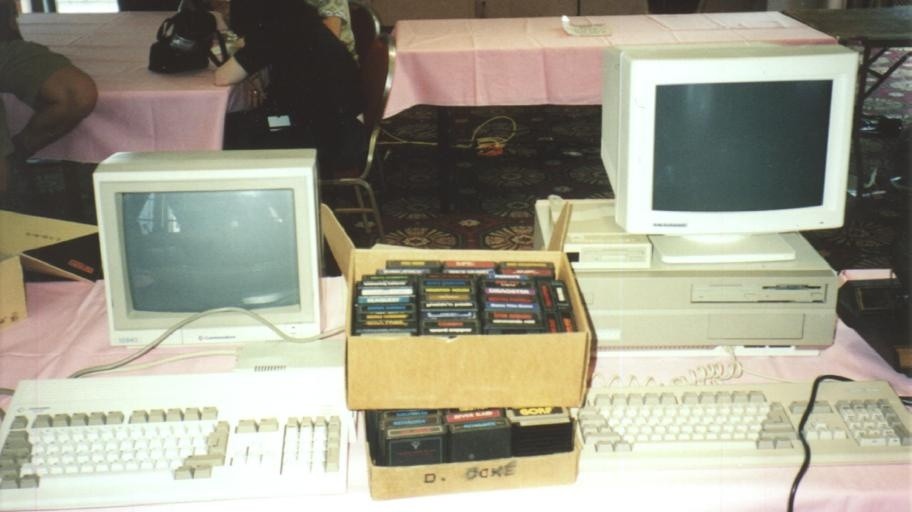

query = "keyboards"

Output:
[[576, 378, 907, 469], [0, 406, 348, 509]]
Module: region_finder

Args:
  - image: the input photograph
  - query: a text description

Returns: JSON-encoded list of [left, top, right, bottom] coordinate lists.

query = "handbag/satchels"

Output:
[[146, 9, 228, 73]]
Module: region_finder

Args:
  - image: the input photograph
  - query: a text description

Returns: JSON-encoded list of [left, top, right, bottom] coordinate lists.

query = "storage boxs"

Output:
[[343, 249, 595, 412], [350, 408, 586, 501], [0, 209, 100, 332]]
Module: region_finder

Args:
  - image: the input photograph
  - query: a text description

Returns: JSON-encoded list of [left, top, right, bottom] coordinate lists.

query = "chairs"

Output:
[[318, 0, 397, 243]]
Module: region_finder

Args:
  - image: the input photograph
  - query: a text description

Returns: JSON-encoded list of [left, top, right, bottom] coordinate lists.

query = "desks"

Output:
[[396, 9, 839, 214], [3, 9, 239, 221], [3, 277, 908, 512]]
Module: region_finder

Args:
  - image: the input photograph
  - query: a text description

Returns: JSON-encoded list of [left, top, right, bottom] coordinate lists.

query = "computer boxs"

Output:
[[533, 197, 840, 356]]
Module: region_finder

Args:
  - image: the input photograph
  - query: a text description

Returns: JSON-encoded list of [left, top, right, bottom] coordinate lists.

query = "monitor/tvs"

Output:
[[91, 146, 319, 351], [591, 25, 875, 261]]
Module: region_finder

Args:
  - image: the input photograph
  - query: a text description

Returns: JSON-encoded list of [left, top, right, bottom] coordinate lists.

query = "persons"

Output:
[[214, 0, 371, 180], [0, 0, 98, 196]]
